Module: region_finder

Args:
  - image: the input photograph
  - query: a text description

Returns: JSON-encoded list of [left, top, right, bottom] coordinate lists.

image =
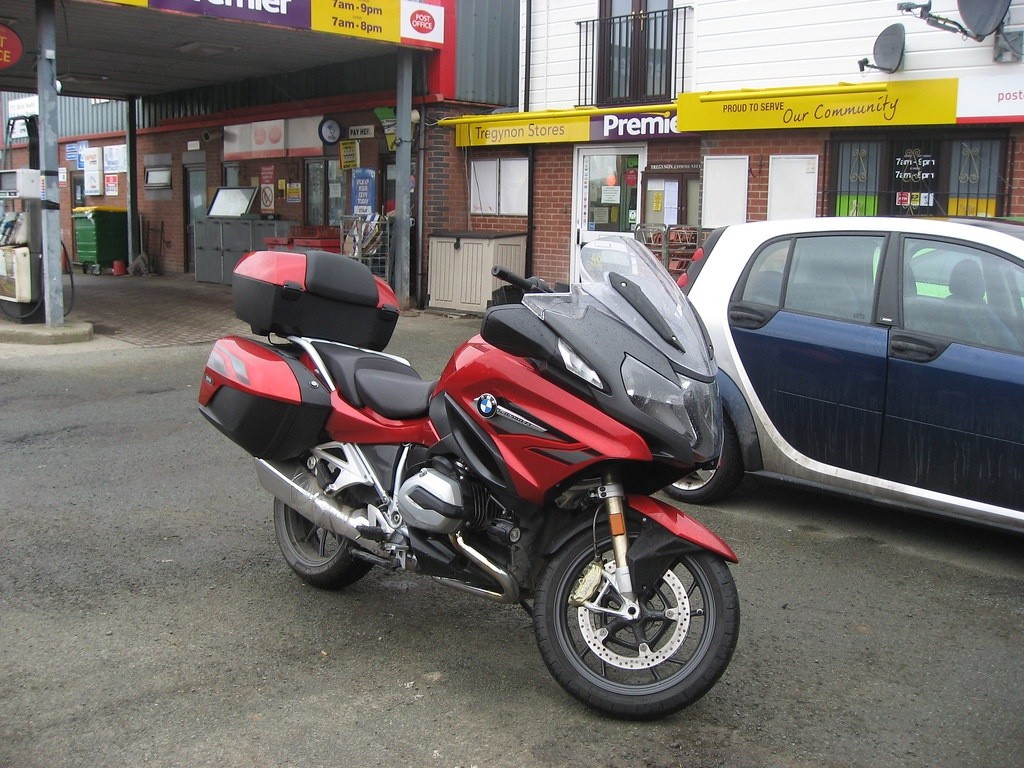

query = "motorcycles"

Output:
[[196, 227, 741, 723]]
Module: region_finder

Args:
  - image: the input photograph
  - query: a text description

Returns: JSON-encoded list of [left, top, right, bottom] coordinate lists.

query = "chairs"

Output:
[[946, 258, 1023, 351], [903, 262, 962, 342]]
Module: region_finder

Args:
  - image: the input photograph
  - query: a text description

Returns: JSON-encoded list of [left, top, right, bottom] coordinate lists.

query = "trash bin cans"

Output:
[[71, 206, 128, 276]]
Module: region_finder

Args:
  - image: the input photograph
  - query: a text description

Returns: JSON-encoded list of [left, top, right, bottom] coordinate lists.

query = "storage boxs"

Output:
[[196, 335, 333, 462]]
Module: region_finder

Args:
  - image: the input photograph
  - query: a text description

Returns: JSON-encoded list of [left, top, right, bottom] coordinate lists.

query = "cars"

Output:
[[652, 214, 1024, 535]]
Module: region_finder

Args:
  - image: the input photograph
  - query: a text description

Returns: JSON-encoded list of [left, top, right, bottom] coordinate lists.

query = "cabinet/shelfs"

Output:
[[633, 222, 703, 282]]
